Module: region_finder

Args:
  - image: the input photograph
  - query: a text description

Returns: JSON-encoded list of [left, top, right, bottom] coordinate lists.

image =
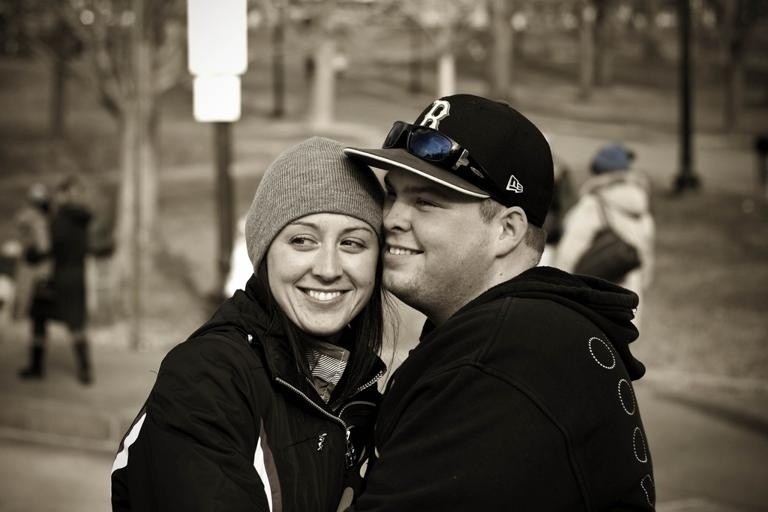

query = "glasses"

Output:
[[382, 120, 497, 190]]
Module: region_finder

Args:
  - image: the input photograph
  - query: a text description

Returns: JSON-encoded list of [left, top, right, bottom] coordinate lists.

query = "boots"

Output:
[[18, 345, 47, 379], [72, 341, 95, 386]]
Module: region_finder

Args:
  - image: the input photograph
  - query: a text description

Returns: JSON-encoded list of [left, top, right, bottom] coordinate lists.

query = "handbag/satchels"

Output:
[[577, 228, 641, 285]]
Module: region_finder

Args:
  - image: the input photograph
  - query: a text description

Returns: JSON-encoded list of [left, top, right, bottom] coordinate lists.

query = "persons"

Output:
[[13, 177, 117, 387], [106, 131, 391, 512], [3, 180, 57, 325], [324, 93, 661, 511], [554, 140, 659, 354]]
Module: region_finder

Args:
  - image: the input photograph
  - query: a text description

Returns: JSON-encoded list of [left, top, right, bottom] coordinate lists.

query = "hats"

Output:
[[590, 145, 632, 175], [343, 92, 556, 230], [244, 134, 385, 275]]
